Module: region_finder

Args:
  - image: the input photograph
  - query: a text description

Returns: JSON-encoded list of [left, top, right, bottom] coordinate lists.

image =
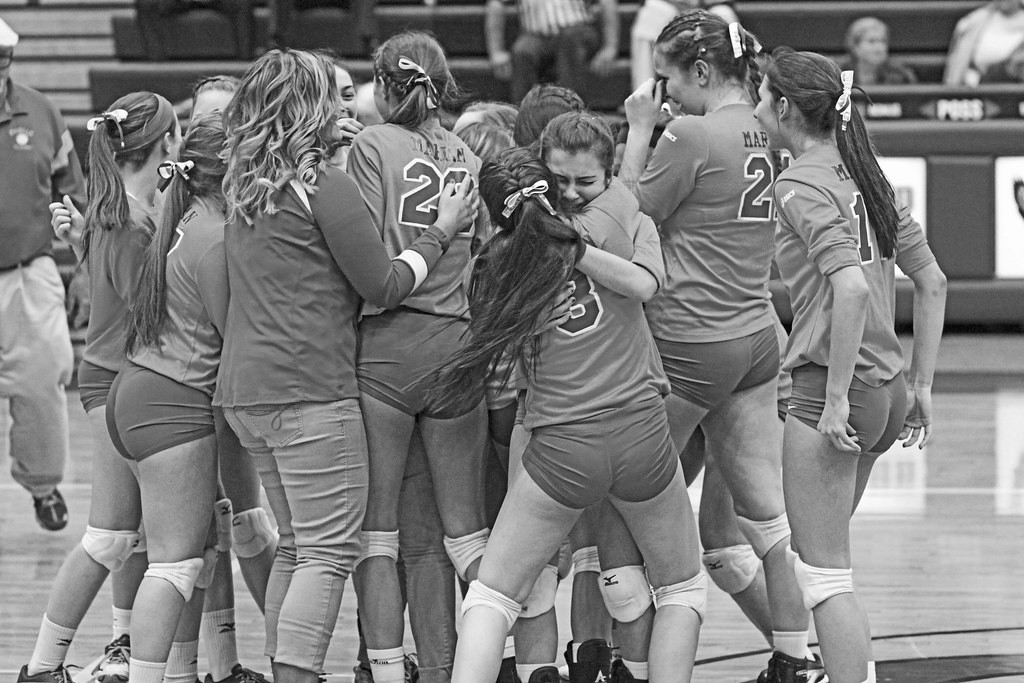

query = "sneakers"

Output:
[[15, 664, 84, 683], [203, 663, 272, 683], [33, 489, 69, 532], [91, 634, 131, 683]]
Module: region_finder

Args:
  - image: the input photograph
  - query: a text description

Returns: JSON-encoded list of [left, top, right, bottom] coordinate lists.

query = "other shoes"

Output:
[[564, 637, 612, 683], [510, 665, 560, 683], [612, 659, 650, 683], [352, 654, 420, 683], [759, 651, 826, 683]]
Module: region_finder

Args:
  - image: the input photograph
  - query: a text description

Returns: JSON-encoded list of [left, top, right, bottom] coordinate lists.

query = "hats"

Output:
[[0, 19, 19, 47]]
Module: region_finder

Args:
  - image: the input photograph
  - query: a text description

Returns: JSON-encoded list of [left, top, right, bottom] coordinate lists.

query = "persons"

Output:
[[838, 16, 924, 117], [0, 16, 91, 532], [16, 0, 948, 683], [942, 0, 1024, 121]]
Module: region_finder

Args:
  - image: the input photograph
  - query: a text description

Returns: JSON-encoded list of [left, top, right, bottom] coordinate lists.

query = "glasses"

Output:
[[0, 51, 14, 71]]
